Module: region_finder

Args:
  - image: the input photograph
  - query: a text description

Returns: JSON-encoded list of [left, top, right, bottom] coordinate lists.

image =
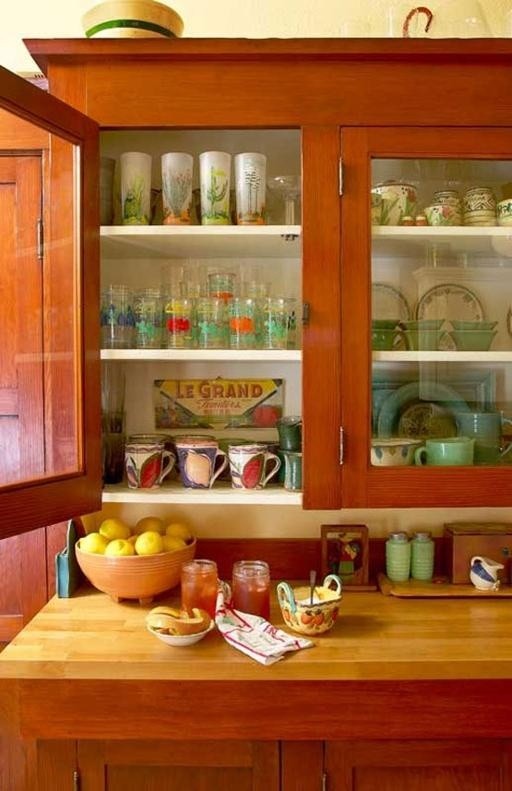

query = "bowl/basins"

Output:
[[276, 574, 344, 636], [146, 618, 217, 649], [424, 186, 512, 225], [74, 532, 198, 598], [370, 317, 498, 352], [371, 178, 424, 226]]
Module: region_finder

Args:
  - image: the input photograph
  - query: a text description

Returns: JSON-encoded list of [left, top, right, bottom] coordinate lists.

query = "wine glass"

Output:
[[266, 172, 304, 225]]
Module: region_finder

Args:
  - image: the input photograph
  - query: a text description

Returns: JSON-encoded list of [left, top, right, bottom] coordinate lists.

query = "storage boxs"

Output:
[[442, 522, 512, 585]]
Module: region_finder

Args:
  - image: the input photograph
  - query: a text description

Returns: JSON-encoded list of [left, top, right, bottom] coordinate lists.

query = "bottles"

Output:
[[385, 530, 412, 582], [409, 532, 436, 584], [231, 561, 273, 627]]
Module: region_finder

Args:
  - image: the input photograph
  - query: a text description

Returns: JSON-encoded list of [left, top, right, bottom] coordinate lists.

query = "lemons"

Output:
[[79, 517, 193, 557]]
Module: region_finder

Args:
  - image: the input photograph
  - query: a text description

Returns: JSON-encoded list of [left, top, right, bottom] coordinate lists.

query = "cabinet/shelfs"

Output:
[[0, 677, 512, 791], [20, 36, 512, 511]]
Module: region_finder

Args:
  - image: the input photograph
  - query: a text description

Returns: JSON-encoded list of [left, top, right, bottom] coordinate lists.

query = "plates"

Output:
[[373, 283, 410, 348], [376, 382, 472, 443], [414, 283, 487, 351]]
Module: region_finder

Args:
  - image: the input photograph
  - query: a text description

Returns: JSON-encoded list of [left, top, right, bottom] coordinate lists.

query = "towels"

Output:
[[212, 579, 314, 665]]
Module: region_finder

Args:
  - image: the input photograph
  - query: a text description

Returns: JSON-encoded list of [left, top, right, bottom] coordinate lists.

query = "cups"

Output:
[[119, 151, 152, 227], [234, 153, 265, 224], [228, 445, 281, 492], [455, 410, 510, 465], [175, 438, 226, 488], [100, 260, 297, 351], [122, 439, 174, 491], [414, 438, 476, 471], [98, 147, 116, 224], [179, 560, 218, 621], [274, 415, 302, 490], [160, 151, 195, 224], [469, 557, 503, 593], [199, 150, 233, 225]]
